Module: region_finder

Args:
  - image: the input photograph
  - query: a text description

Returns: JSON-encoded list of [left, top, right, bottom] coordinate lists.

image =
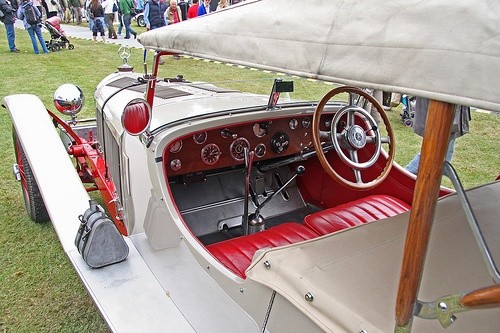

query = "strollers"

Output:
[[41, 16, 74, 52]]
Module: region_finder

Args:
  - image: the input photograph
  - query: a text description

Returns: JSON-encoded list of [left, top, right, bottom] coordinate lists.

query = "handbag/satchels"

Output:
[[0, 10, 5, 19], [51, 0, 56, 5], [130, 8, 137, 17]]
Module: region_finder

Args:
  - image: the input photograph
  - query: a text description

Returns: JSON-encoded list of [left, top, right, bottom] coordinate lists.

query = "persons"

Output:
[[352, 86, 385, 131], [0, 0, 21, 54], [404, 97, 471, 178], [17, 0, 50, 54], [0, 0, 246, 42]]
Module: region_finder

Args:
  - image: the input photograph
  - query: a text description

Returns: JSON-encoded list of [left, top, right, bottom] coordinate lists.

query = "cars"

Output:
[[0, 0, 500, 333], [132, 11, 146, 28]]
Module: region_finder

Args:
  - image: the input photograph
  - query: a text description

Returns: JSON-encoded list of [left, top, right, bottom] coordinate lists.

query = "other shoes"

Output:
[[10, 49, 20, 53], [124, 37, 129, 39], [108, 37, 112, 38], [47, 50, 51, 53], [134, 33, 137, 39], [112, 38, 117, 39]]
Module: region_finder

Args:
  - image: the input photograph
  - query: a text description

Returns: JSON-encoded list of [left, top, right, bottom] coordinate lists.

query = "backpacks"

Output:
[[24, 2, 37, 25]]
